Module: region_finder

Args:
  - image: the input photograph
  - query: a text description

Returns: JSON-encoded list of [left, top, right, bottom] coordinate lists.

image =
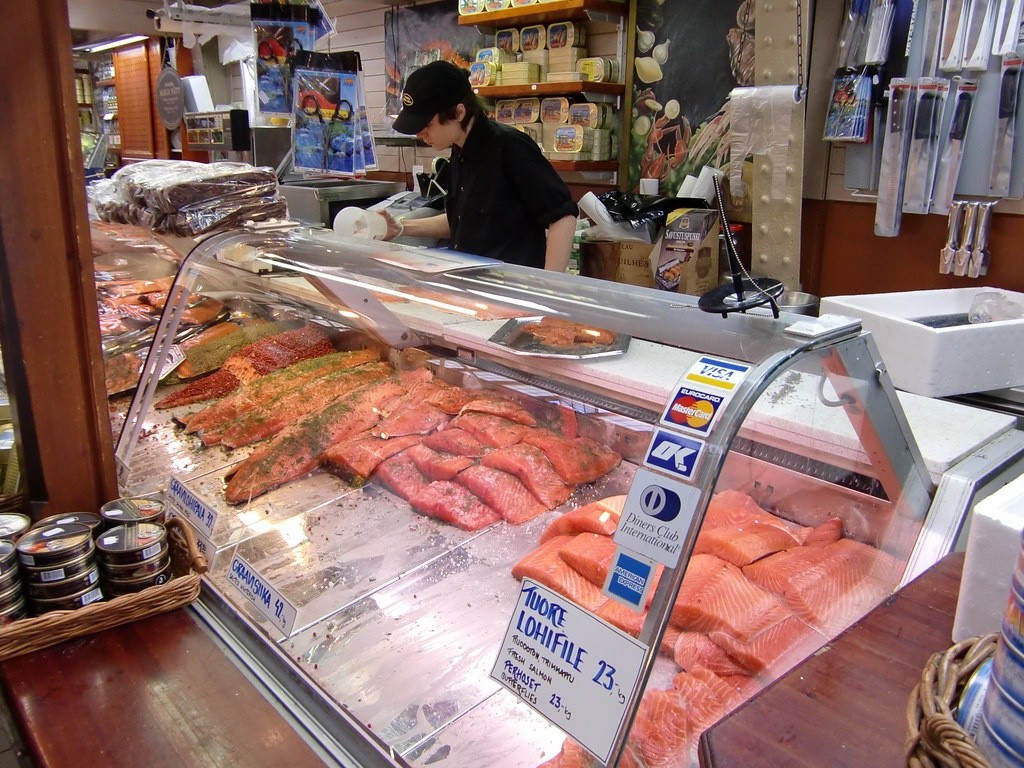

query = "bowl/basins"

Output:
[[760, 290, 819, 315]]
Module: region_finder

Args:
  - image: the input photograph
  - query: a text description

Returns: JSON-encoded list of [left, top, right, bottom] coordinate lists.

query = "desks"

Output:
[[698, 553, 963, 768]]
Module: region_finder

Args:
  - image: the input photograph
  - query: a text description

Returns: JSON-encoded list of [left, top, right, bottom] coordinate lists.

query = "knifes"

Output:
[[989, 66, 1021, 198], [922, 0, 1015, 77], [882, 86, 972, 232]]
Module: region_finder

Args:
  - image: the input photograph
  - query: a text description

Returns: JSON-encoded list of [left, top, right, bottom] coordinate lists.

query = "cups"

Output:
[[334, 206, 387, 241]]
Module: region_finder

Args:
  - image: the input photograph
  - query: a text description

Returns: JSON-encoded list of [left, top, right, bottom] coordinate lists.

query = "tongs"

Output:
[[938, 201, 995, 277]]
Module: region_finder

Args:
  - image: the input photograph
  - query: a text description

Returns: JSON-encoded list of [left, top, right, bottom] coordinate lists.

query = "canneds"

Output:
[[0, 498, 172, 632]]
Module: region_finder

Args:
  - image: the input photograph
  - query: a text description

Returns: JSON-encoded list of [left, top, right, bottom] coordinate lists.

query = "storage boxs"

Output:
[[818, 285, 1024, 397], [579, 208, 719, 298], [953, 473, 1024, 644]]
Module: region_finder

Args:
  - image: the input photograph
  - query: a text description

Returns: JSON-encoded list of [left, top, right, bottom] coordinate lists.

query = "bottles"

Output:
[[720, 225, 747, 274]]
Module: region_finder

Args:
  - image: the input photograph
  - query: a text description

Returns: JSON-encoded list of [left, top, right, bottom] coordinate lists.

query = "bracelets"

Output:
[[388, 218, 404, 242]]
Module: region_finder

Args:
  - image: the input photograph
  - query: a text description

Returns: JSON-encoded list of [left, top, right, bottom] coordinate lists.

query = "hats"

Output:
[[392, 60, 467, 135]]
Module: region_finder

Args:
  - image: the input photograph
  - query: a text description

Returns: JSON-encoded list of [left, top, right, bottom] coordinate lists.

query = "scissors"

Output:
[[258, 38, 302, 113], [833, 89, 856, 137], [302, 95, 353, 173]]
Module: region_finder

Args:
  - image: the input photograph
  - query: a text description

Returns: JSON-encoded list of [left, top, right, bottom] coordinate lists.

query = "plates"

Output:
[[485, 316, 632, 360]]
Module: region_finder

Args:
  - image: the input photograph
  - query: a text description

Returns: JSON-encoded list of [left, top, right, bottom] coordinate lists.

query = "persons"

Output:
[[378, 60, 579, 273]]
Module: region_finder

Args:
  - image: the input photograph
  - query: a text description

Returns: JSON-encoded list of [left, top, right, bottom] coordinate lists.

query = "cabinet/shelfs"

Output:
[[94, 35, 209, 169], [457, 1, 636, 192]]
